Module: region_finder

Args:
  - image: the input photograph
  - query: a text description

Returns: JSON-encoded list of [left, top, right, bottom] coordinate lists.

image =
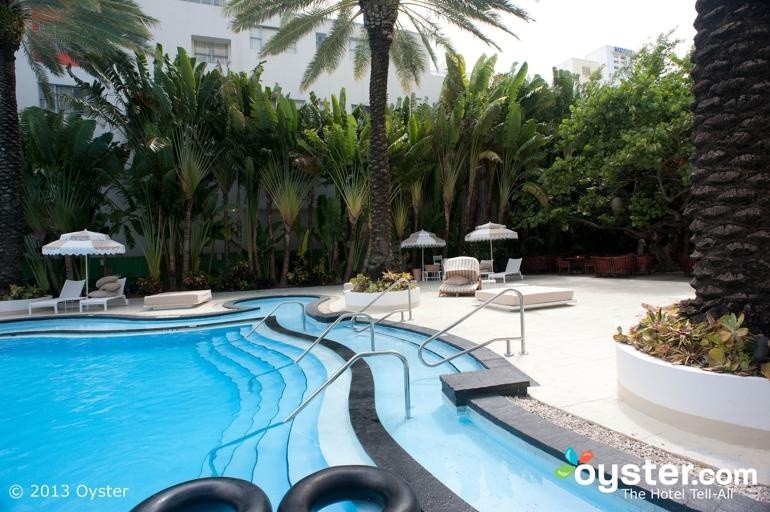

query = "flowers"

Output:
[[615, 299, 768, 374], [350, 269, 417, 292]]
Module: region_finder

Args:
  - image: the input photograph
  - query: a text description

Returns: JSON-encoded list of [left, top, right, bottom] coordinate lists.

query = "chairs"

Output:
[[28, 276, 129, 316], [522, 253, 651, 277], [423, 255, 523, 296], [676, 249, 696, 277]]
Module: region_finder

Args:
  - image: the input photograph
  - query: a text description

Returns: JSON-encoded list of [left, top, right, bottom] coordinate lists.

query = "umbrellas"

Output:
[[400, 228, 447, 281], [41, 228, 126, 310], [465, 220, 519, 272]]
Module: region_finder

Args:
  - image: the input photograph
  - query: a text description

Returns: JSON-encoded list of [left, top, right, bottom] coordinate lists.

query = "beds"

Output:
[[143, 289, 213, 310], [475, 284, 577, 312]]
